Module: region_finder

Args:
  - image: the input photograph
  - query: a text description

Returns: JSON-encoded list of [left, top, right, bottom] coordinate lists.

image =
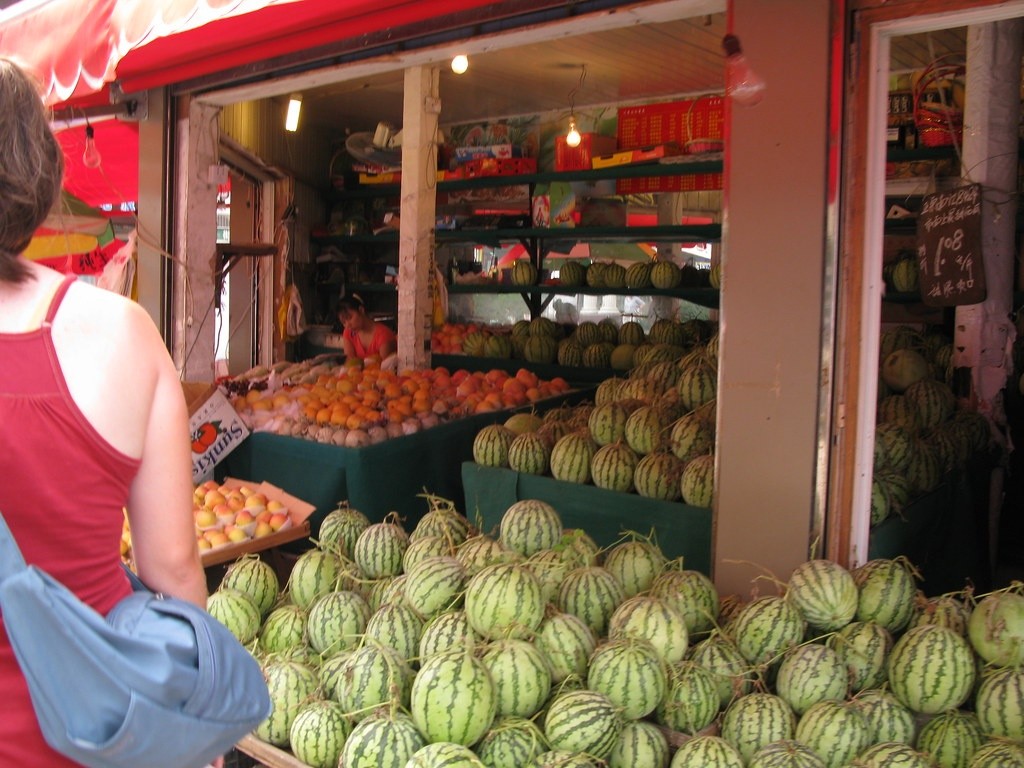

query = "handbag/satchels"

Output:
[[0, 565, 271, 768]]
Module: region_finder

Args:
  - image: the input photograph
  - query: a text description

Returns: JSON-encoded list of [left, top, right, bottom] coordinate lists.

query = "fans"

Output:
[[345, 119, 403, 168]]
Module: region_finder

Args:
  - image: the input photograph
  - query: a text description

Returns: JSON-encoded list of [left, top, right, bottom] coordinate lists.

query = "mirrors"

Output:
[[332, 171, 723, 336]]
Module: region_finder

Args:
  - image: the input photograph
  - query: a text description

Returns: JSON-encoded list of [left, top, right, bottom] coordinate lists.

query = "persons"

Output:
[[623, 295, 645, 322], [337, 291, 394, 365], [0, 59, 207, 768]]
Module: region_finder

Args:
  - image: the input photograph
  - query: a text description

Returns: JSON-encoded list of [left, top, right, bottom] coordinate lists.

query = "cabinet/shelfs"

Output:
[[302, 141, 963, 385]]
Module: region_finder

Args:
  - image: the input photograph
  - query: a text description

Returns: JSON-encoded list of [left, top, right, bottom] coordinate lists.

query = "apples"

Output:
[[232, 368, 568, 448], [431, 319, 486, 356], [121, 481, 289, 573]]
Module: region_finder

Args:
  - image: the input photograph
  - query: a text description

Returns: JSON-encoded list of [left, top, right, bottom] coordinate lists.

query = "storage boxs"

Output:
[[181, 381, 251, 485], [353, 143, 537, 184], [531, 195, 626, 227], [555, 134, 683, 171]]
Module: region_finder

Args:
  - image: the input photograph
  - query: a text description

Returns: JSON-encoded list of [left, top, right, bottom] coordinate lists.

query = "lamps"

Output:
[[82, 125, 102, 168], [566, 116, 581, 148], [285, 94, 304, 132], [451, 55, 469, 74], [722, 33, 768, 109]]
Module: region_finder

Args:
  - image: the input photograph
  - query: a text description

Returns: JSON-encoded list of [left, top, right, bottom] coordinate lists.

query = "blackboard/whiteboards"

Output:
[[916, 183, 988, 309]]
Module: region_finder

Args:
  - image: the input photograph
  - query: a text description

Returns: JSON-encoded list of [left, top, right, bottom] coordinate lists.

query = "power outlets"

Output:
[[208, 163, 228, 184]]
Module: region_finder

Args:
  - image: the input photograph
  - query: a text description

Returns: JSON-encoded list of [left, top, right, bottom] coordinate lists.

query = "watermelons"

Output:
[[475, 324, 989, 528], [458, 257, 918, 370], [206, 496, 1024, 768]]
